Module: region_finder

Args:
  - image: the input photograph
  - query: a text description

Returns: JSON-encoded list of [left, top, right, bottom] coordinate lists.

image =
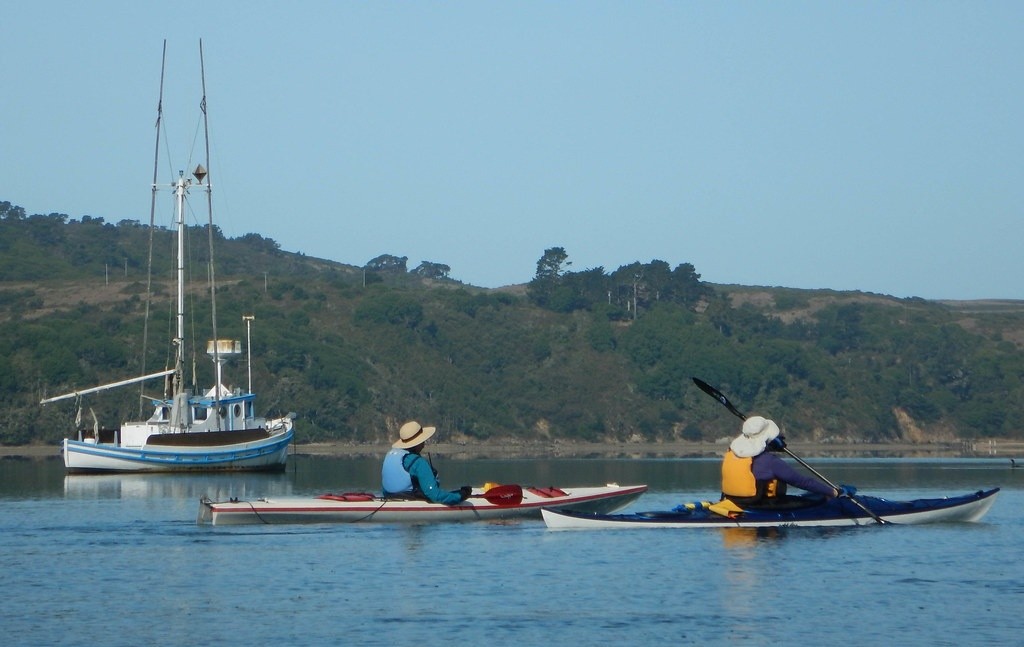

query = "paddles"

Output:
[[343, 483, 524, 508], [690, 376, 892, 525]]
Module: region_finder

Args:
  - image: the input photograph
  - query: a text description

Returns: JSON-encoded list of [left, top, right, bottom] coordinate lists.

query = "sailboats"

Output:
[[37, 35, 302, 475]]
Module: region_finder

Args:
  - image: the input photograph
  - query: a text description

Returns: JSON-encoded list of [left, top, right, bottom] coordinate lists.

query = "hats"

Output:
[[391, 420, 436, 448], [730, 415, 779, 457]]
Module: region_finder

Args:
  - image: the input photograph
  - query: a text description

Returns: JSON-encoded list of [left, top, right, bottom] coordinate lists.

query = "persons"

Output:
[[382, 422, 472, 505], [721, 415, 843, 511]]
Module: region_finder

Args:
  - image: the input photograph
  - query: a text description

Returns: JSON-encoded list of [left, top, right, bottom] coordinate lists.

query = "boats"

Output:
[[540, 486, 1002, 531], [198, 486, 647, 525]]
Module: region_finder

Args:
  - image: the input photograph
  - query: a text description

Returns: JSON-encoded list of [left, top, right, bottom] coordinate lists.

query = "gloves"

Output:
[[835, 484, 857, 499], [768, 434, 787, 451], [459, 485, 472, 501]]
